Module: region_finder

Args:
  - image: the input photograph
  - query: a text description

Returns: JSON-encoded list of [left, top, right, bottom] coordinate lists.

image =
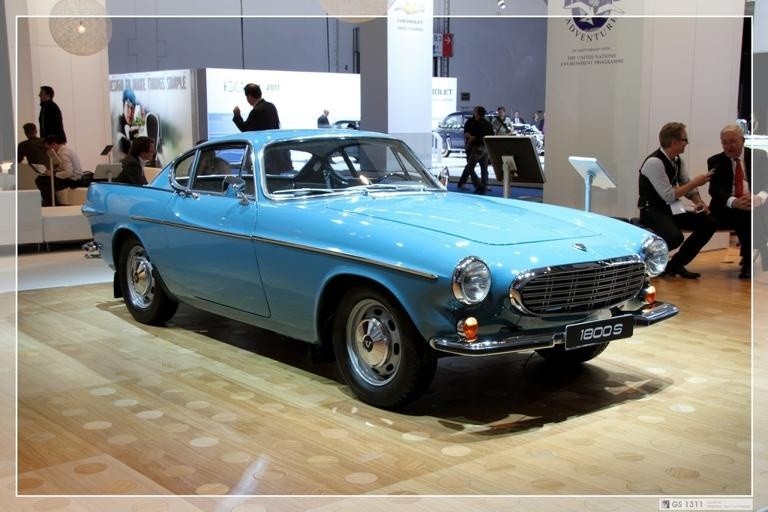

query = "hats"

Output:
[[123, 89, 136, 106]]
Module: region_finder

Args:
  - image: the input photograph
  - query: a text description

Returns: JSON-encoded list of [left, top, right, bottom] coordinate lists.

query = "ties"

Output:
[[671, 160, 679, 186], [732, 159, 745, 198]]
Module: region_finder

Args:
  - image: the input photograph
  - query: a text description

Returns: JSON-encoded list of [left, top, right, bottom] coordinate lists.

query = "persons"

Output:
[[18, 123, 46, 164], [232, 83, 279, 132], [112, 137, 156, 185], [707, 125, 768, 278], [457, 106, 544, 195], [318, 111, 337, 164], [188, 139, 230, 175], [638, 123, 717, 279], [35, 135, 82, 206], [116, 89, 160, 150], [39, 86, 66, 145]]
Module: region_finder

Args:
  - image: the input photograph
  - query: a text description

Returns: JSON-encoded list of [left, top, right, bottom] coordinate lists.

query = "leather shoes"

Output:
[[665, 259, 701, 280]]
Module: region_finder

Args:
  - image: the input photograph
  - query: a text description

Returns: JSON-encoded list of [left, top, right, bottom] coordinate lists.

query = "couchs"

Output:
[[0, 161, 93, 255], [143, 166, 161, 184]]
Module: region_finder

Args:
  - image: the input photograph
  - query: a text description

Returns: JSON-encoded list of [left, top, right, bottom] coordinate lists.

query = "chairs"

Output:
[[196, 157, 232, 193], [306, 157, 341, 190]]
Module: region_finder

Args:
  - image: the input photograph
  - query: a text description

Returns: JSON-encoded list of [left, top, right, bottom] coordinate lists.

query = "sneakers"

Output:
[[759, 245, 768, 272], [738, 262, 755, 281], [457, 184, 494, 195]]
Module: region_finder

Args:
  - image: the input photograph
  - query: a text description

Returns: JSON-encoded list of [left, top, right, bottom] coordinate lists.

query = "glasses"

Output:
[[666, 135, 690, 144]]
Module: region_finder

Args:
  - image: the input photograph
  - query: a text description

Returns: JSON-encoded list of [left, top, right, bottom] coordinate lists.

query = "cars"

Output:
[[82, 127, 683, 416], [331, 119, 360, 133], [432, 111, 543, 157]]
